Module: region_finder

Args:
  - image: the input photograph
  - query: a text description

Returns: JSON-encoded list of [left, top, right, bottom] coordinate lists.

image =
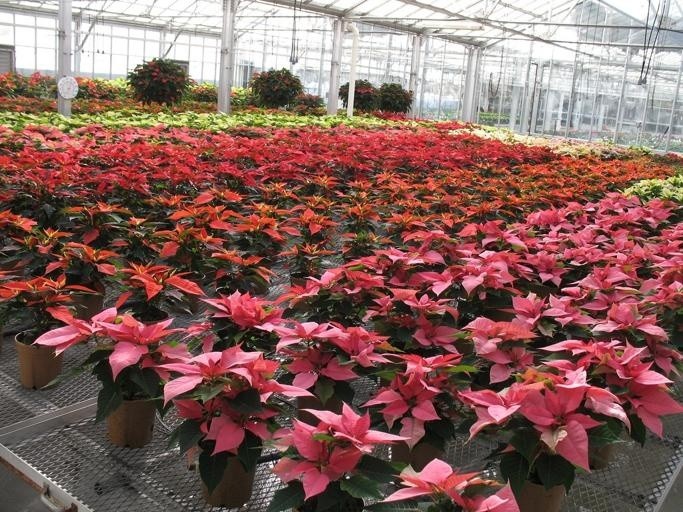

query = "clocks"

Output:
[[57, 76, 79, 100]]
